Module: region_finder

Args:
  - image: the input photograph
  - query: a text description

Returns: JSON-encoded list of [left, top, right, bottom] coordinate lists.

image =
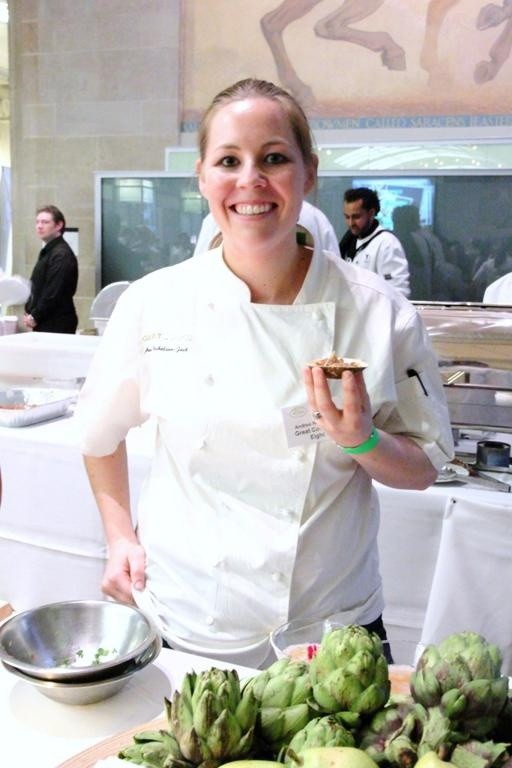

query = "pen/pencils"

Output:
[[407, 369, 430, 399]]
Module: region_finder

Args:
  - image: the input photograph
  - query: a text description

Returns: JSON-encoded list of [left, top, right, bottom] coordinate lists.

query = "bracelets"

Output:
[[336, 424, 379, 454]]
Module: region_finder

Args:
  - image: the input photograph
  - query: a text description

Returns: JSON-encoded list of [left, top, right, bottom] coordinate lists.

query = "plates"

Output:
[[435, 464, 470, 484]]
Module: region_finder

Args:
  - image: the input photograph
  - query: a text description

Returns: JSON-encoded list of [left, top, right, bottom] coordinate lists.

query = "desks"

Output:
[[0, 414, 263, 766]]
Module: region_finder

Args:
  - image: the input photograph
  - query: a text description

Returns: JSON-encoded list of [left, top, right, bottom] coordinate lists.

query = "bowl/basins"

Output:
[[0, 599, 164, 706]]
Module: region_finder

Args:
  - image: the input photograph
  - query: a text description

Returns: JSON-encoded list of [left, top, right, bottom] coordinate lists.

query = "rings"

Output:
[[311, 411, 320, 419]]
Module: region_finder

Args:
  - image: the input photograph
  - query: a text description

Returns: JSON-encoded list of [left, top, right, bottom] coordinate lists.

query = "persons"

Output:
[[72, 78, 456, 676], [390, 204, 511, 307], [24, 205, 80, 333], [338, 186, 411, 298], [102, 211, 195, 284]]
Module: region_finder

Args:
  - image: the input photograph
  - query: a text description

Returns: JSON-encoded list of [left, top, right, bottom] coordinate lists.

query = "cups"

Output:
[[267, 617, 345, 660]]
